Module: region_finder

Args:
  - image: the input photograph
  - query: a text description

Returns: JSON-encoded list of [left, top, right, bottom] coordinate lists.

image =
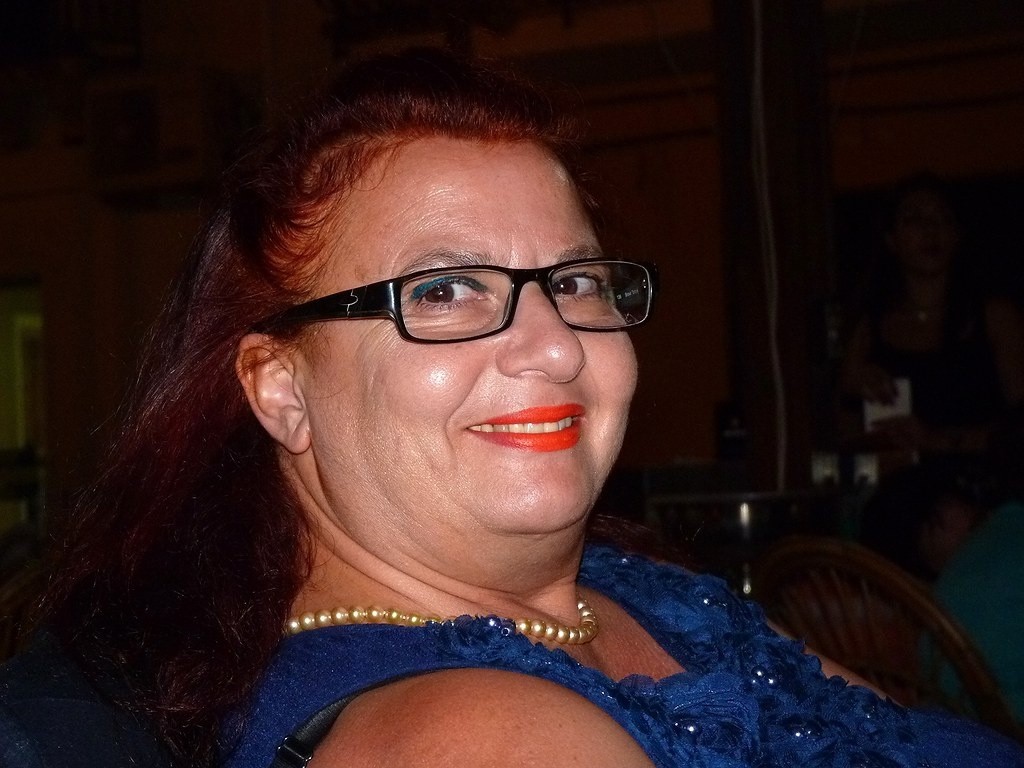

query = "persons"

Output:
[[0, 97, 1024, 768], [833, 182, 1024, 715]]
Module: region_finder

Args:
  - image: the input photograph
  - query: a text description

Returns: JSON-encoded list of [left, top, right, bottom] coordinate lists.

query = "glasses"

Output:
[[247, 256, 654, 344]]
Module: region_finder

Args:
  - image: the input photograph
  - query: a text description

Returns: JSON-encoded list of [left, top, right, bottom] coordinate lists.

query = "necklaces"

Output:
[[286, 600, 600, 645]]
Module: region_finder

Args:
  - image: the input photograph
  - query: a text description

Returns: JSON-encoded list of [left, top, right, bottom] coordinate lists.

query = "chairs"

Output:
[[0, 651, 165, 768], [744, 534, 1024, 745]]
